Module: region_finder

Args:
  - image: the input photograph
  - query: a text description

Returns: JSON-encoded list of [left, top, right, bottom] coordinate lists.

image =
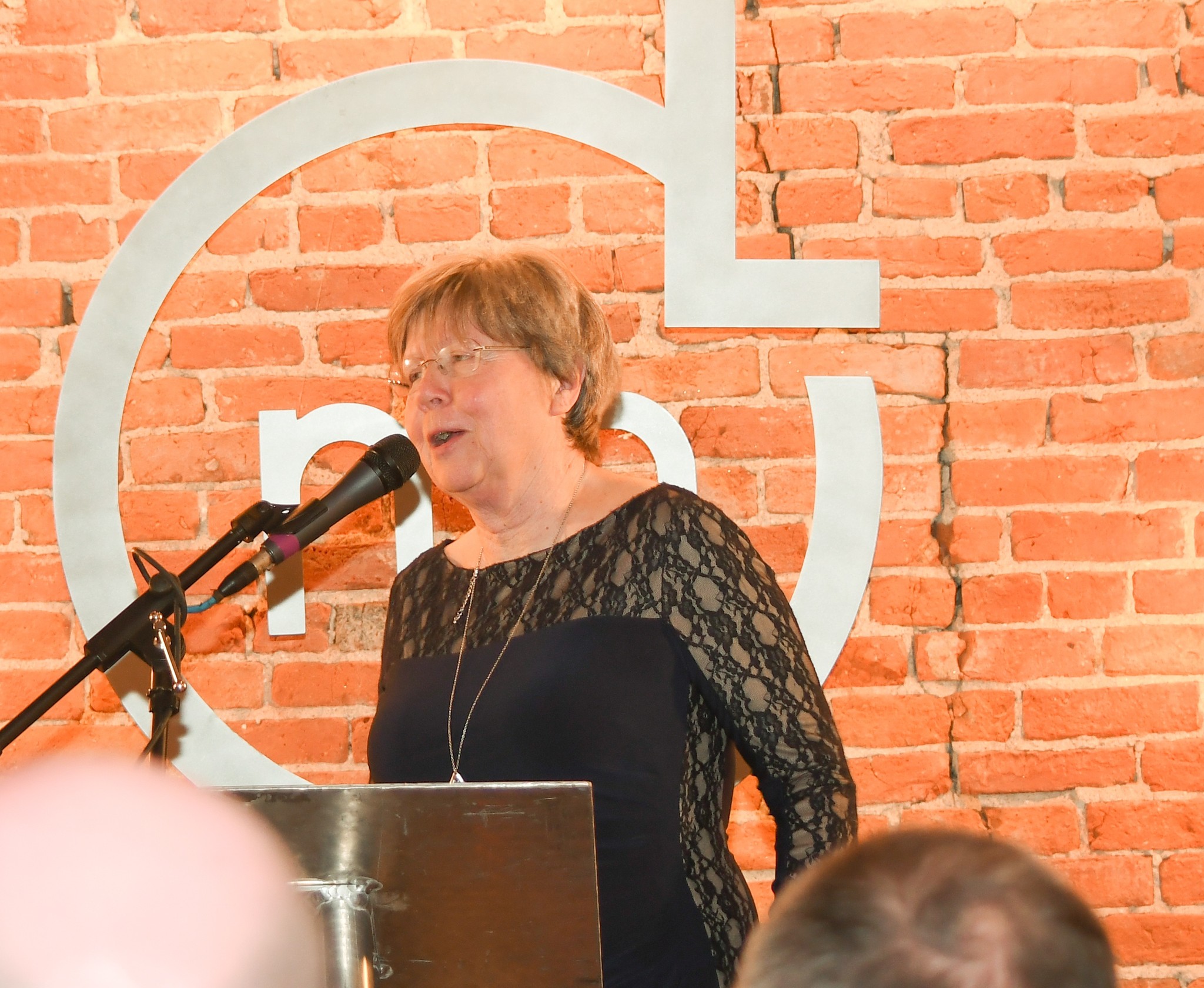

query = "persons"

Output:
[[0, 741, 328, 988], [365, 252, 859, 988], [736, 829, 1113, 988]]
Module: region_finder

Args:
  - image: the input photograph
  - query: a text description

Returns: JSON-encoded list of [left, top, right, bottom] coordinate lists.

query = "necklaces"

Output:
[[444, 466, 585, 784]]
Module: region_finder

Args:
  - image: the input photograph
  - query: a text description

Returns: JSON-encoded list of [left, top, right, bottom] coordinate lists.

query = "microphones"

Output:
[[215, 433, 421, 595]]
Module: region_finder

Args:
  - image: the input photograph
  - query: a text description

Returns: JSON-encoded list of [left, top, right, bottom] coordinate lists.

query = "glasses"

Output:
[[388, 338, 541, 397]]
